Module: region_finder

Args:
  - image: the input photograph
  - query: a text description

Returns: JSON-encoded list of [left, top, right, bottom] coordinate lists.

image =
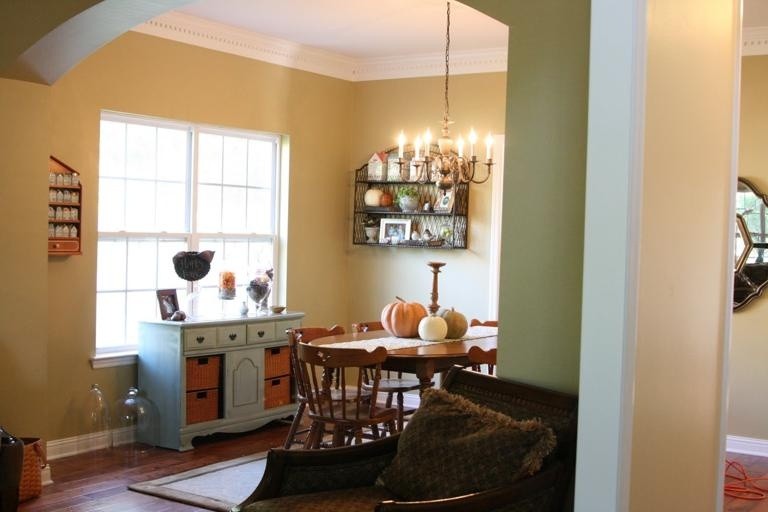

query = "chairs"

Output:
[[350, 321, 437, 438], [466, 345, 497, 376], [295, 339, 398, 449], [468, 318, 497, 377], [225, 363, 579, 512], [282, 324, 375, 447]]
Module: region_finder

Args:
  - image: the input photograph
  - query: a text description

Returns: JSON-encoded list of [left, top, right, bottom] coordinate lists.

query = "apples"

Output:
[[381, 194, 392, 207]]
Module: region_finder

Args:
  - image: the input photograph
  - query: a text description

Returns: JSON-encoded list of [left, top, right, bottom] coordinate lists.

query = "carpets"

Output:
[[126, 418, 409, 512]]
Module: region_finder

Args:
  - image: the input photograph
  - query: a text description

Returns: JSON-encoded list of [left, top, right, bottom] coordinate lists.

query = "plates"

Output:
[[361, 206, 398, 212], [404, 239, 442, 247]]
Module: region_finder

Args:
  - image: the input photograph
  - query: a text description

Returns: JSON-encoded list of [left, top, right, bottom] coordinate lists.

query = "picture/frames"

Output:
[[156, 288, 181, 320], [377, 216, 411, 245], [431, 187, 459, 214]]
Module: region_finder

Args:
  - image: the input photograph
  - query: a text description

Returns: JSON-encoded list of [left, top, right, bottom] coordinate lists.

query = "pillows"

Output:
[[366, 387, 560, 502]]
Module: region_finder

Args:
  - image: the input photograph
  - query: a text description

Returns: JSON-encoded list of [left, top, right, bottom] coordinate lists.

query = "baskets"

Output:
[[17, 435, 49, 502]]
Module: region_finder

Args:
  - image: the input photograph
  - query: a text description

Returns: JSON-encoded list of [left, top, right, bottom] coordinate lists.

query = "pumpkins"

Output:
[[418, 314, 448, 341], [364, 189, 383, 206], [440, 308, 468, 338], [380, 302, 428, 337]]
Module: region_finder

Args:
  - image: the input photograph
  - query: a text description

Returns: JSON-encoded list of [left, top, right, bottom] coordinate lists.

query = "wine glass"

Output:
[[244, 286, 270, 317], [364, 227, 379, 243], [441, 224, 452, 247]]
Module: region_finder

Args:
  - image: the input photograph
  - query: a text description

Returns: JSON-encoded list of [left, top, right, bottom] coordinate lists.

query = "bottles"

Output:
[[411, 230, 420, 240], [422, 229, 431, 241], [239, 302, 248, 314]]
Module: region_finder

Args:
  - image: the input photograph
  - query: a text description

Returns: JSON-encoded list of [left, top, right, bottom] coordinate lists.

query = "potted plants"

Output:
[[391, 186, 423, 214], [360, 214, 382, 244]]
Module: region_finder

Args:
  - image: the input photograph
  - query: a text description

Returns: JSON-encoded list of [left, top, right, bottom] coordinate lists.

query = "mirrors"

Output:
[[730, 173, 768, 313]]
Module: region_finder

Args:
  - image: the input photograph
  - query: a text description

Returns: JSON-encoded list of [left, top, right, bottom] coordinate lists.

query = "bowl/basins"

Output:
[[270, 306, 286, 313]]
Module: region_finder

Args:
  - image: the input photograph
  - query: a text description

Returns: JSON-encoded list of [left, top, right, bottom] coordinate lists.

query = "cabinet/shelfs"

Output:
[[349, 143, 470, 251], [49, 155, 85, 256], [132, 307, 305, 455]]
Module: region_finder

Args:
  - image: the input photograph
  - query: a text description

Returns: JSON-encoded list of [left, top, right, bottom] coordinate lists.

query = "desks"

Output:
[[308, 324, 497, 398]]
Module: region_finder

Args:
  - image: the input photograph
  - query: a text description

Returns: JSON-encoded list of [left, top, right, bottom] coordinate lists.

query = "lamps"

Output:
[[390, 1, 496, 196]]
